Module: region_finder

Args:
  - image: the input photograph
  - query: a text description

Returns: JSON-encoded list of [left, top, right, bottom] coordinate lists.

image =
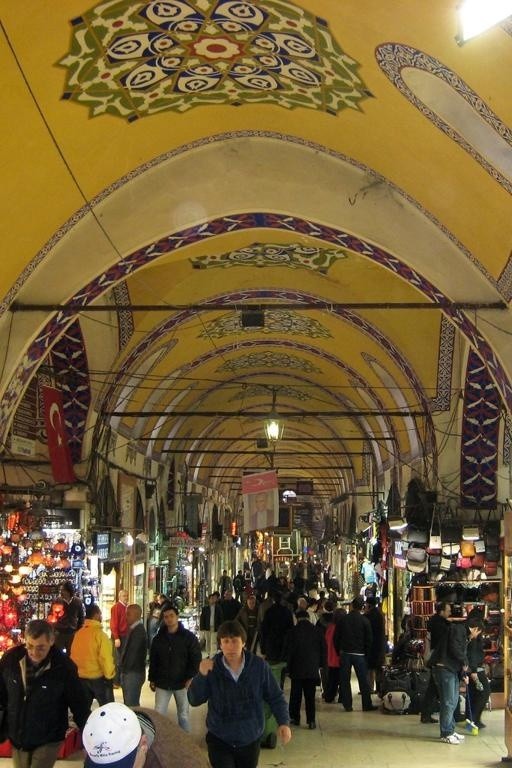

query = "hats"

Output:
[[81, 702, 143, 768]]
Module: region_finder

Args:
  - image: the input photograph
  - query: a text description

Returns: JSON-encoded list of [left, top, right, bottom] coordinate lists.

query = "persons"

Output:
[[421, 602, 465, 723], [200, 556, 387, 730], [467, 609, 492, 730], [185, 619, 293, 768], [427, 618, 484, 745], [0, 578, 210, 767], [249, 491, 274, 530]]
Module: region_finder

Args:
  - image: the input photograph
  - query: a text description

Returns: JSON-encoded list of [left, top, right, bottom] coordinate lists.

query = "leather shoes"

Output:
[[420, 715, 438, 723], [454, 713, 466, 721]]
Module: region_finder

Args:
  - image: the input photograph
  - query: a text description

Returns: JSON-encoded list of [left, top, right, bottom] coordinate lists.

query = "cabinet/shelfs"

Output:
[[410, 579, 505, 710]]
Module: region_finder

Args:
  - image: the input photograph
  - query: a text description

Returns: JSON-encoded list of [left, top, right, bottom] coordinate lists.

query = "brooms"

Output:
[[456, 672, 479, 736]]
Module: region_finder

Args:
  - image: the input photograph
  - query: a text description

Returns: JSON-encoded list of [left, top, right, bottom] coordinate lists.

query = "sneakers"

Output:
[[452, 732, 464, 740], [441, 735, 460, 744]]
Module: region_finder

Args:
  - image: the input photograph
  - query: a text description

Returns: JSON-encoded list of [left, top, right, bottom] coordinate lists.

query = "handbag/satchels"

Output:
[[380, 639, 439, 716], [505, 511, 511, 556], [390, 519, 505, 576], [436, 584, 500, 615]]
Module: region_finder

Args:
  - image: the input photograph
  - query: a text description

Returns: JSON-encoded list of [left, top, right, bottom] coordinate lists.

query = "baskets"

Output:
[[411, 575, 433, 639]]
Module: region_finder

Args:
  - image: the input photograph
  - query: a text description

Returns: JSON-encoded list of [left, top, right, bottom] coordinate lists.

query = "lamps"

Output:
[[455, 1, 512, 47], [261, 390, 286, 442]]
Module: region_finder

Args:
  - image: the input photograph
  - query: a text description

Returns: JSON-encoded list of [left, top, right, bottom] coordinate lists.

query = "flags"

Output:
[[42, 386, 77, 484]]
